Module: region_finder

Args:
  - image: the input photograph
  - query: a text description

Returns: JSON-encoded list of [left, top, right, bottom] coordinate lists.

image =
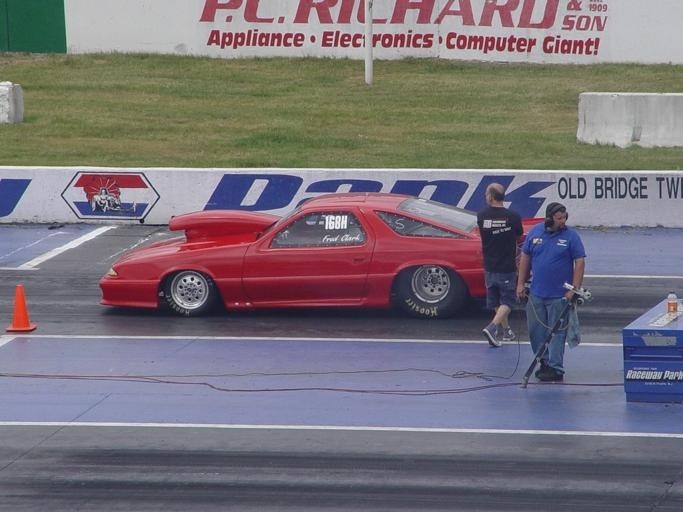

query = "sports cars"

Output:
[[93, 187, 545, 325]]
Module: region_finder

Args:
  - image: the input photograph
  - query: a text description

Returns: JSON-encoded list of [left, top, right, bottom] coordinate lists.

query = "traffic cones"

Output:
[[2, 282, 38, 332]]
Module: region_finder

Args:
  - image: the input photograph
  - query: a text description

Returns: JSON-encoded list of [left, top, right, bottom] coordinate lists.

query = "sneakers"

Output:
[[534, 364, 564, 381], [502, 325, 516, 341], [482, 321, 502, 348]]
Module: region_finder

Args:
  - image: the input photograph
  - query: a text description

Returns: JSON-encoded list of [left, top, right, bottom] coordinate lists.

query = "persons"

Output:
[[475, 183, 525, 348], [515, 203, 587, 382]]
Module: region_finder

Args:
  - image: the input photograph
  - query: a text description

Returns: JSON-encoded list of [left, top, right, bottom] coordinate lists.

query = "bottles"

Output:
[[667, 290, 678, 321]]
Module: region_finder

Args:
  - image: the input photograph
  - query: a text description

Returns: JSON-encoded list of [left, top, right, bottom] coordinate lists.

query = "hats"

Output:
[[546, 201, 566, 217]]
